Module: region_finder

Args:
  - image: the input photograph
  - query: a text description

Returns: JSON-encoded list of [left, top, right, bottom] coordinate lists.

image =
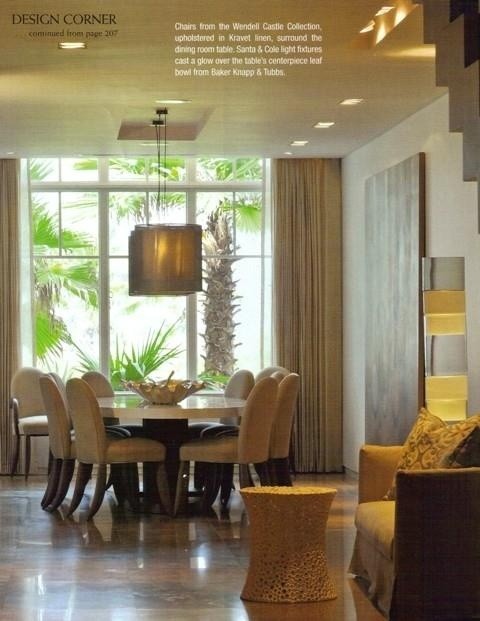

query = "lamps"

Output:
[[420, 256, 468, 422], [127, 109, 202, 297]]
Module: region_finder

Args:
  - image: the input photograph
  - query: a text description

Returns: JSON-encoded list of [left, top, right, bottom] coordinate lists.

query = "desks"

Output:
[[96, 394, 245, 512]]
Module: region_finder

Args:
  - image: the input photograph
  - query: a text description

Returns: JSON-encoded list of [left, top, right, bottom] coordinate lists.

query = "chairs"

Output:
[[268, 371, 284, 384], [79, 370, 143, 437], [186, 370, 255, 515], [172, 376, 278, 514], [64, 377, 172, 521], [36, 373, 130, 511], [255, 364, 291, 378], [200, 374, 300, 512], [344, 443, 480, 620], [10, 368, 48, 481]]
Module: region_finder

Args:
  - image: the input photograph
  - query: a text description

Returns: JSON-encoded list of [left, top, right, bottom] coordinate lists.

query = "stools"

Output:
[[239, 484, 338, 604]]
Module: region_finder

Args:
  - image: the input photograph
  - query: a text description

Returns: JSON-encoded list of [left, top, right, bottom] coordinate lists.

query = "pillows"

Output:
[[379, 407, 480, 500]]
